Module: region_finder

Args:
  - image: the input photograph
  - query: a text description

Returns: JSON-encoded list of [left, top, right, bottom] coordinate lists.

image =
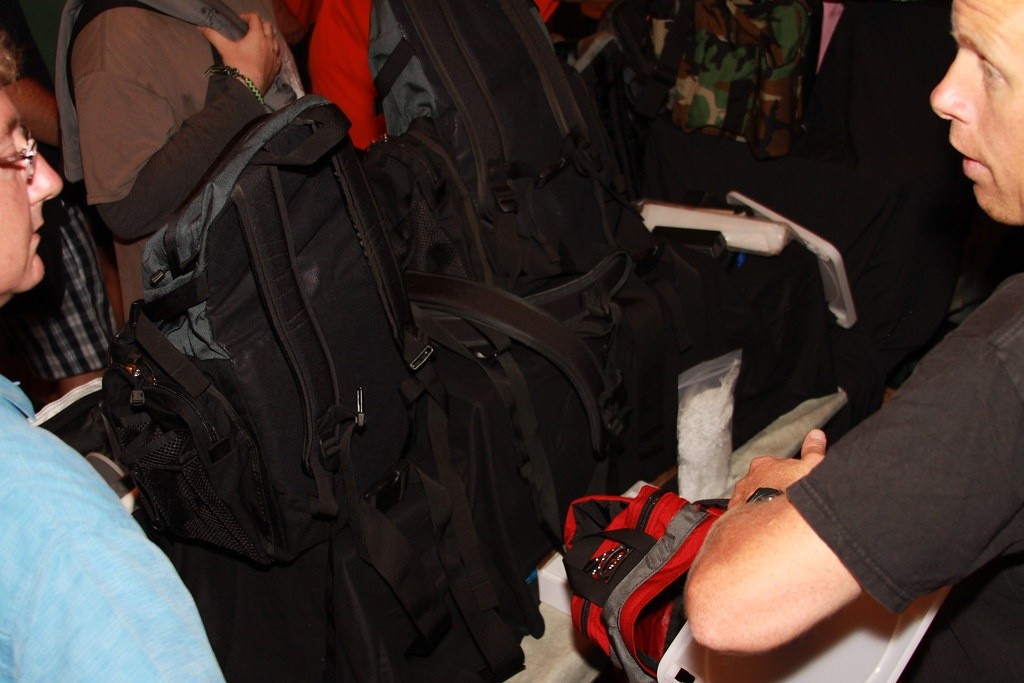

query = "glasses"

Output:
[[0, 124, 37, 180]]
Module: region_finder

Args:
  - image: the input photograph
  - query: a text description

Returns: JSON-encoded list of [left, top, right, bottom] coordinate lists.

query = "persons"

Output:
[[684, 0, 1024, 682], [0, 0, 1024, 435], [0, 85, 225, 683]]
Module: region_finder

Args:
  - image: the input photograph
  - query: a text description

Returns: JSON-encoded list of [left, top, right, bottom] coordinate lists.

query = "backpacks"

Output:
[[95, 3, 967, 683]]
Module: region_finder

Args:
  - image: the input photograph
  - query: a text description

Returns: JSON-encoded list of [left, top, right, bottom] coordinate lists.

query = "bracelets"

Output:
[[205, 66, 264, 106], [747, 488, 784, 503]]
